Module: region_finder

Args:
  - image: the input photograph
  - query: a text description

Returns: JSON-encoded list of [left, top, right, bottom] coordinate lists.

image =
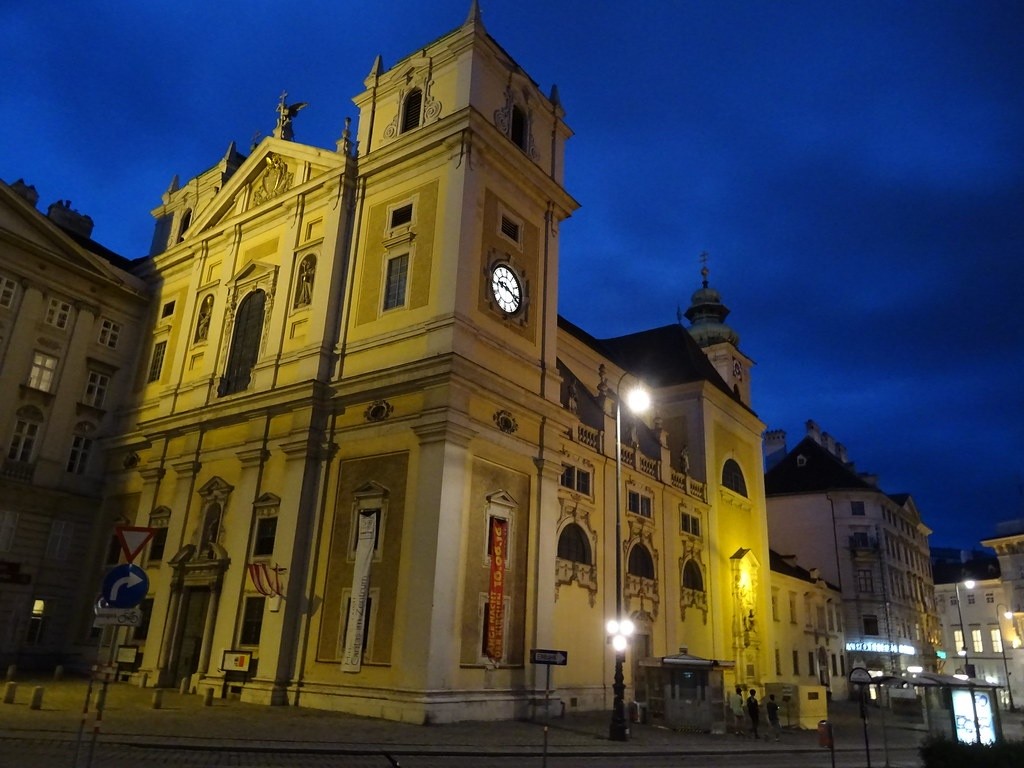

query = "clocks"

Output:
[[732, 358, 741, 380], [489, 264, 523, 315]]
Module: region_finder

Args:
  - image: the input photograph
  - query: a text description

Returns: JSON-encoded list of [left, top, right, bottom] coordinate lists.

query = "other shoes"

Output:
[[755, 735, 760, 739], [776, 737, 783, 742]]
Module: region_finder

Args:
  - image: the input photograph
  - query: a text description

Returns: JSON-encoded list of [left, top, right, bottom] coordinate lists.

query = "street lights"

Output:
[[609, 370, 653, 742], [955, 578, 977, 676], [996, 603, 1016, 713]]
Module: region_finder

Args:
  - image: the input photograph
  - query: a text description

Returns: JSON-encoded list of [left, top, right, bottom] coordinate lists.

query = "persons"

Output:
[[747, 689, 760, 739], [730, 687, 745, 735], [825, 683, 832, 716], [763, 695, 782, 743]]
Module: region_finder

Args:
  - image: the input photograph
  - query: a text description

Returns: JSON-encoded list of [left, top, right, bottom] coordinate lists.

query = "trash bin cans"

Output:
[[632, 699, 647, 723], [818, 719, 833, 748]]
